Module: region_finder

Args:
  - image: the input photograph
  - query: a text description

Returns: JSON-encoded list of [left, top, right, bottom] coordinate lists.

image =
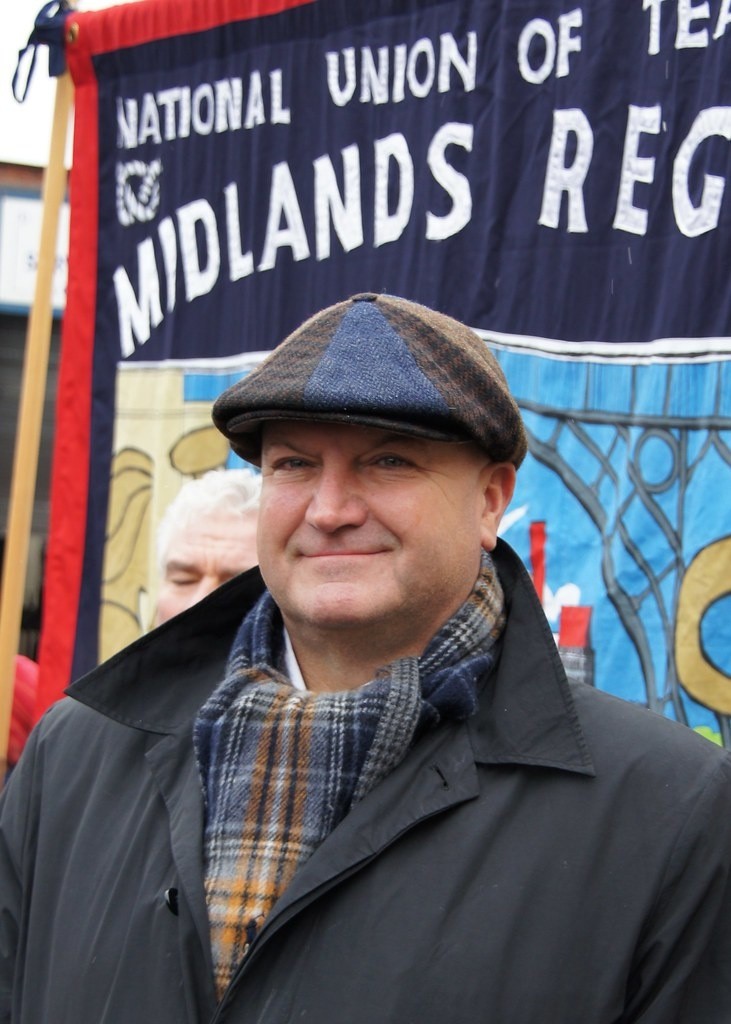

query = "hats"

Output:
[[211, 294, 528, 469]]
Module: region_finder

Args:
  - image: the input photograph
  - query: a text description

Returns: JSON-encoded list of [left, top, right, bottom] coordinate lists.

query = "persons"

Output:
[[156, 468, 262, 622], [0, 294, 731, 1024]]
